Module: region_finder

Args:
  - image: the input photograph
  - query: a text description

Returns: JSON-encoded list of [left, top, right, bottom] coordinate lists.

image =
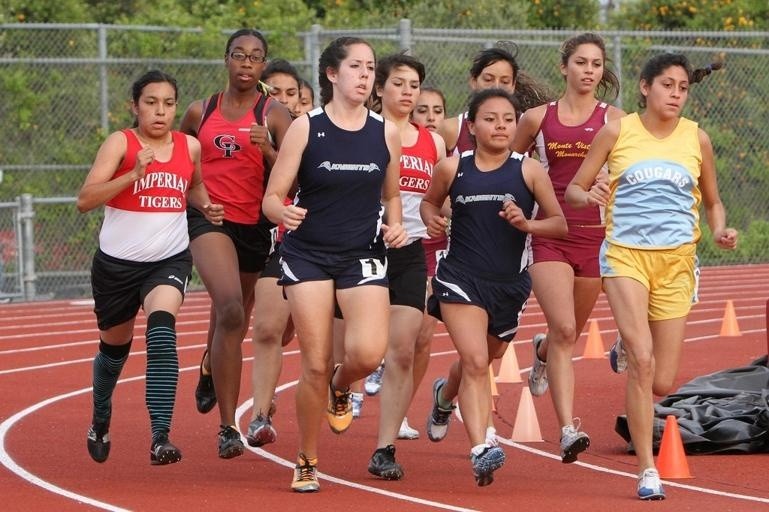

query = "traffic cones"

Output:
[[653, 414, 695, 481], [718, 301, 740, 339], [583, 319, 608, 361], [487, 361, 500, 399], [512, 388, 547, 442], [497, 338, 524, 384], [490, 400, 497, 414]]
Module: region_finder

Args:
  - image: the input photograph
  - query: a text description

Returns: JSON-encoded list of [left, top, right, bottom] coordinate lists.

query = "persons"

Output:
[[563, 54, 741, 500], [418, 90, 568, 486], [262, 38, 406, 493], [349, 34, 627, 480], [177, 29, 316, 459], [75, 71, 227, 465]]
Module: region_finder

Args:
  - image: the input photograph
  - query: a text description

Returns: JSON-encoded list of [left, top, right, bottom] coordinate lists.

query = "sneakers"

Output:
[[426, 377, 457, 442], [216, 424, 244, 459], [195, 347, 217, 414], [290, 452, 321, 493], [326, 363, 354, 435], [609, 329, 629, 374], [246, 411, 278, 448], [86, 420, 111, 463], [150, 433, 182, 466], [559, 417, 590, 464], [397, 416, 419, 440], [367, 444, 404, 479], [470, 446, 505, 487], [364, 363, 384, 396], [637, 468, 666, 500], [351, 392, 364, 418], [528, 333, 550, 397]]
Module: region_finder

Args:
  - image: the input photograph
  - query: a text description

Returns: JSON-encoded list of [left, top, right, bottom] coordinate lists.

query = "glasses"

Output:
[[225, 49, 268, 64]]
